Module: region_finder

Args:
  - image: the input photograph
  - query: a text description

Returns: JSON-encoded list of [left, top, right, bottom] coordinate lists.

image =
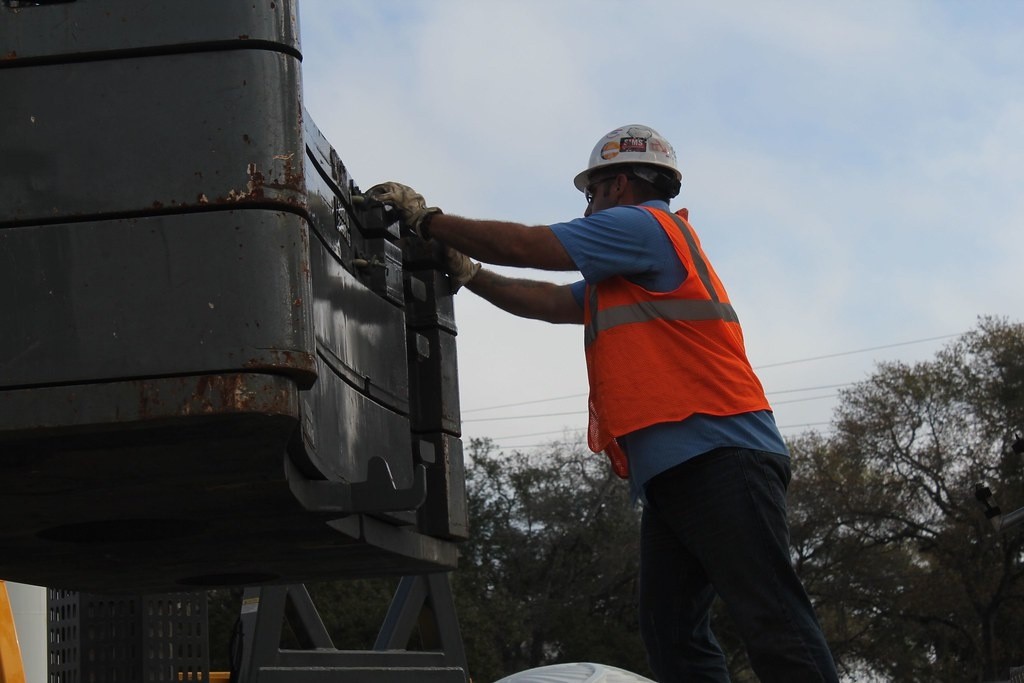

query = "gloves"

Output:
[[363, 181, 440, 243], [415, 239, 483, 295]]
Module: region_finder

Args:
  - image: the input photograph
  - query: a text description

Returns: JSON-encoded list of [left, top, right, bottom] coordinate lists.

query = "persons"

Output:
[[364, 124, 840, 683]]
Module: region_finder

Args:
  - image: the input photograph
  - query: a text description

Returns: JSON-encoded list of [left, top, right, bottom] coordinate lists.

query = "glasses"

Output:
[[584, 177, 613, 202]]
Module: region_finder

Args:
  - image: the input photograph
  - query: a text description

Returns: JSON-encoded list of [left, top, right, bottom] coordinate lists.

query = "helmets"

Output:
[[574, 125, 682, 197]]
